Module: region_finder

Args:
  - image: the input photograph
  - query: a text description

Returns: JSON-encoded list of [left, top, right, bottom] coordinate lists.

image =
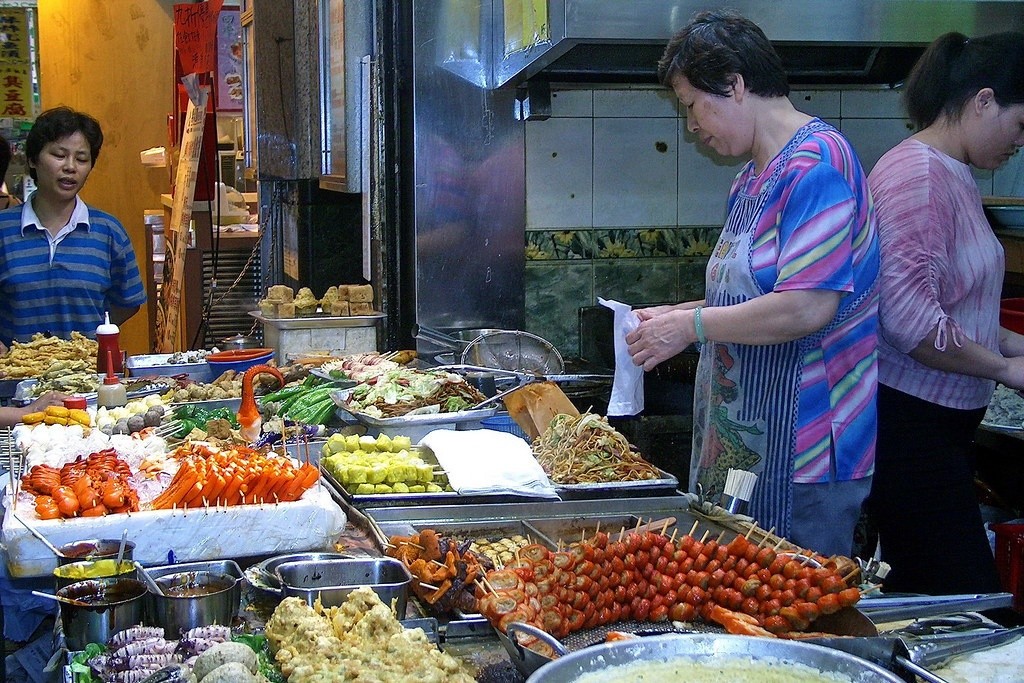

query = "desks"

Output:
[[262, 325, 378, 368]]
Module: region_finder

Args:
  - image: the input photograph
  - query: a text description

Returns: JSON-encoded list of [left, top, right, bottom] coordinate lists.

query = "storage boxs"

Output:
[[207, 347, 275, 361], [209, 351, 276, 382], [989, 522, 1024, 616], [329, 390, 500, 444]]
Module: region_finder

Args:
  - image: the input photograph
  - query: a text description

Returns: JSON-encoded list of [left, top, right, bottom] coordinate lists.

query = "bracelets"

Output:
[[694, 306, 709, 344]]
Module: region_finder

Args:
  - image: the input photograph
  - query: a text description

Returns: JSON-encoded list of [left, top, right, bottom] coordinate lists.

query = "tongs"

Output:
[[879, 625, 1024, 667], [424, 365, 535, 409], [855, 592, 1013, 623]]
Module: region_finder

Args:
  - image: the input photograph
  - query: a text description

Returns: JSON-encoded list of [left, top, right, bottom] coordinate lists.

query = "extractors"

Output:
[[492, 0, 1024, 123]]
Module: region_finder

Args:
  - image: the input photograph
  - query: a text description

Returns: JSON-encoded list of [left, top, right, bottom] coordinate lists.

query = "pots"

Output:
[[493, 553, 878, 681], [523, 634, 906, 683]]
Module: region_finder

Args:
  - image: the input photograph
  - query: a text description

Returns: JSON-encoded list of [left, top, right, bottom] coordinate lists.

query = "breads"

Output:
[[259, 284, 375, 319]]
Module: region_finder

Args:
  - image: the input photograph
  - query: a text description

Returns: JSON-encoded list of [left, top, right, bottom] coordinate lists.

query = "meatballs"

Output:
[[95, 393, 165, 437]]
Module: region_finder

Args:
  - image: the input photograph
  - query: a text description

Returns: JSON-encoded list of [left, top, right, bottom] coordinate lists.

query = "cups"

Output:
[[221, 330, 258, 348], [720, 492, 748, 515]]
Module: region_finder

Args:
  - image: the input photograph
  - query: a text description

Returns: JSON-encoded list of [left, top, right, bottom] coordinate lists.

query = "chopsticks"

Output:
[[724, 468, 758, 502]]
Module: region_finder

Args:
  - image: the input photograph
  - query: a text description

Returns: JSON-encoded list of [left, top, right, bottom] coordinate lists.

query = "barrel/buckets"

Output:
[[998, 298, 1024, 336]]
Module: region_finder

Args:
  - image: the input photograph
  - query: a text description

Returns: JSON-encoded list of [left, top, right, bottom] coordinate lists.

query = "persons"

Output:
[[0, 107, 143, 346], [627, 13, 878, 559], [0, 391, 74, 430], [867, 32, 1023, 596]]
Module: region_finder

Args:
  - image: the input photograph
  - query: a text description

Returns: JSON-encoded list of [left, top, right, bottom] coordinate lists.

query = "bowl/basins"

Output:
[[245, 563, 283, 596], [56, 577, 147, 651], [147, 571, 237, 641], [205, 348, 276, 379], [258, 553, 358, 588], [989, 206, 1024, 229], [54, 559, 138, 591], [57, 538, 137, 567]]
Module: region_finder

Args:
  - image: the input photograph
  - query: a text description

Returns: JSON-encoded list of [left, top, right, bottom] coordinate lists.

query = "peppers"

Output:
[[172, 374, 339, 440]]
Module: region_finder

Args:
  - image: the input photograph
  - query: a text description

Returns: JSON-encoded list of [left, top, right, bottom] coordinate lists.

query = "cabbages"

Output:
[[543, 414, 657, 481], [348, 368, 475, 419]]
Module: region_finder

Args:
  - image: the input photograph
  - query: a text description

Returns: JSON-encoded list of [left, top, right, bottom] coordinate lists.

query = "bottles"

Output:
[[96, 312, 123, 373], [97, 351, 127, 410]]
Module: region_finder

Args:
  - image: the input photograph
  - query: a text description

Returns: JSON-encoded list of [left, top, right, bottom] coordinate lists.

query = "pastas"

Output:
[[529, 414, 662, 483]]
